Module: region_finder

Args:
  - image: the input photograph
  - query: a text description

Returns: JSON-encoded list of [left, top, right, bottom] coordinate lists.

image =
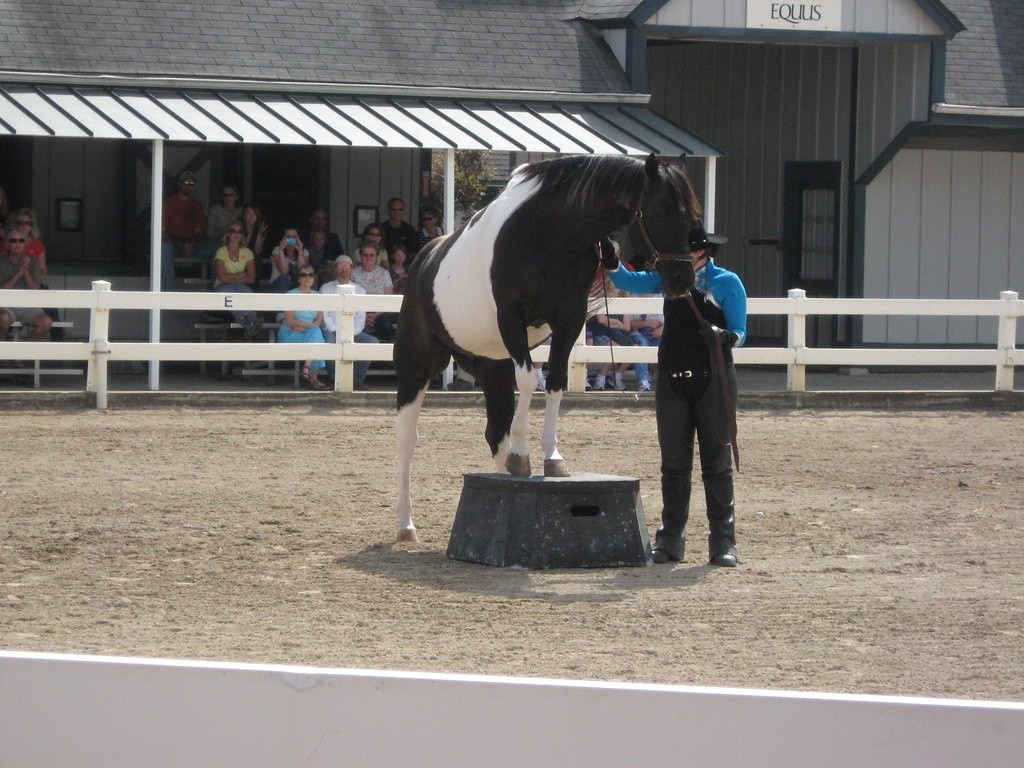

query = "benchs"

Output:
[[0, 306, 85, 392], [179, 255, 479, 391]]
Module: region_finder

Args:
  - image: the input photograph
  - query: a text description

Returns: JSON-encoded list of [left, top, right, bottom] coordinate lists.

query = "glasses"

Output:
[[8, 238, 27, 244], [176, 169, 440, 281], [15, 219, 35, 226]]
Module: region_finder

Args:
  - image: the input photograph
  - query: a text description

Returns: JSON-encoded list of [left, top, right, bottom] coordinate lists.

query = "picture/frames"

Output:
[[353, 205, 379, 238], [58, 198, 84, 233]]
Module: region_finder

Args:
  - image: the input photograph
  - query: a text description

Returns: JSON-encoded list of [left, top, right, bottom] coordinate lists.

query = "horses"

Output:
[[393, 151, 696, 543]]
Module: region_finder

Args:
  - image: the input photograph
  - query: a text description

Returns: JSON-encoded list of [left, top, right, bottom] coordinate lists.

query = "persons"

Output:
[[0, 188, 64, 368], [605, 226, 747, 566], [534, 242, 663, 390], [163, 174, 206, 278], [208, 184, 476, 391]]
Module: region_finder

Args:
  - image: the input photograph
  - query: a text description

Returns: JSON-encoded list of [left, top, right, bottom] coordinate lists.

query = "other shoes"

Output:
[[429, 379, 440, 389], [307, 380, 327, 390], [12, 359, 23, 367], [353, 380, 369, 391], [301, 365, 311, 381], [651, 549, 679, 564], [0, 360, 10, 366], [584, 379, 593, 391], [447, 379, 473, 391], [712, 554, 737, 568]]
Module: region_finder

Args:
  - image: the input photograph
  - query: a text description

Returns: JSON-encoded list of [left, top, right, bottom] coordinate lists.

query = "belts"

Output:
[[665, 368, 710, 379]]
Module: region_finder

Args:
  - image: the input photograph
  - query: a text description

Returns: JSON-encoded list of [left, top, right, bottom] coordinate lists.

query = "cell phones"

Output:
[[285, 237, 297, 245]]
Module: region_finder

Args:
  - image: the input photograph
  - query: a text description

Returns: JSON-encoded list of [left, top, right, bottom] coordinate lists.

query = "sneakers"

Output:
[[608, 374, 626, 391], [638, 379, 650, 391], [593, 374, 605, 390], [537, 375, 546, 390]]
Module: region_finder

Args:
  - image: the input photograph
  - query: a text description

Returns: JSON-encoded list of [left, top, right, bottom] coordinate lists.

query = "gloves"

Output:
[[698, 320, 737, 348], [601, 238, 619, 271]]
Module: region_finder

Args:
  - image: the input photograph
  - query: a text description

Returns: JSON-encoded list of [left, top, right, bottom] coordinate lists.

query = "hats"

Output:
[[688, 219, 729, 252], [174, 171, 197, 187], [333, 255, 354, 268]]
